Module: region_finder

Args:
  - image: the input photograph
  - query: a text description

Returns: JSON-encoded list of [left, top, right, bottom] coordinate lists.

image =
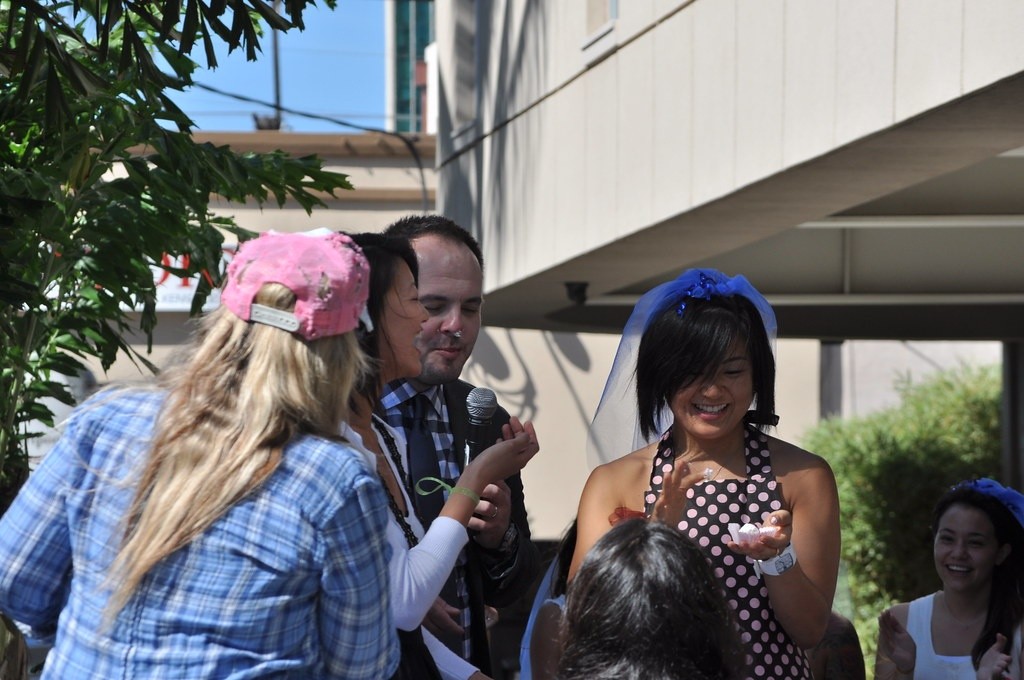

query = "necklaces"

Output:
[[676, 446, 732, 481], [943, 600, 987, 631]]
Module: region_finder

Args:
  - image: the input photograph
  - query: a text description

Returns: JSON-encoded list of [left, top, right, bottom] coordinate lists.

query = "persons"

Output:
[[806, 612, 868, 680], [567, 268, 842, 679], [347, 229, 539, 680], [563, 518, 745, 680], [380, 213, 536, 680], [0, 229, 399, 680], [872, 475, 1024, 680], [481, 516, 578, 680]]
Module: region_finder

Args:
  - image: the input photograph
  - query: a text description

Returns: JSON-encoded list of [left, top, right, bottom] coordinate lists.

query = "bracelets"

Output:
[[416, 477, 481, 505], [897, 664, 914, 675]]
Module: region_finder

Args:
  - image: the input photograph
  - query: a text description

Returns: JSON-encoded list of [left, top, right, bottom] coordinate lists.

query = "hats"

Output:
[[222, 226, 374, 341]]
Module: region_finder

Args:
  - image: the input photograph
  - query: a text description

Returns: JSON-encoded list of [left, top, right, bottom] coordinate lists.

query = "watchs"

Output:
[[498, 522, 519, 554], [752, 541, 797, 580]]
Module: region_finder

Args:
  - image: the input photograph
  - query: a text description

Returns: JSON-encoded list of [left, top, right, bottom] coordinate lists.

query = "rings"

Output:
[[777, 549, 779, 555], [490, 506, 498, 518]]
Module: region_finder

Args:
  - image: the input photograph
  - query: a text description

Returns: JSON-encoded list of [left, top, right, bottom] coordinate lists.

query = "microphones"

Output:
[[462, 389, 498, 471]]
